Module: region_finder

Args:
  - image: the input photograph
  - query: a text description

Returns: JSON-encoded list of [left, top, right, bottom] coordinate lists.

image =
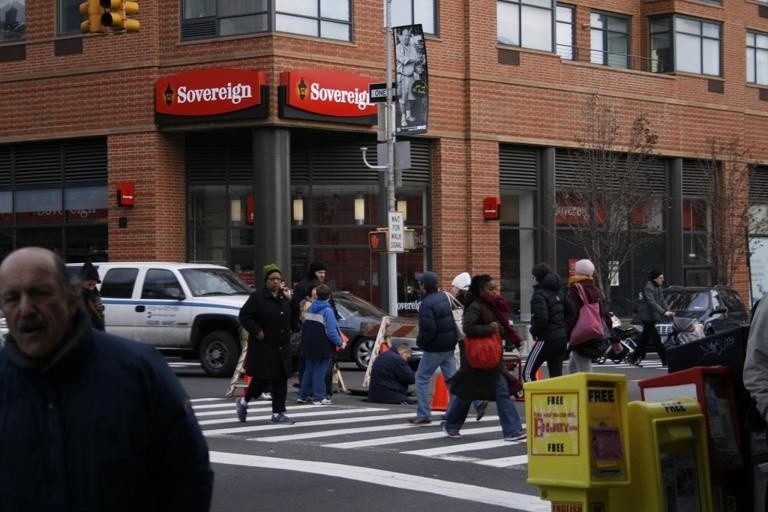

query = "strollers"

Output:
[[590, 305, 646, 365], [663, 311, 710, 351]]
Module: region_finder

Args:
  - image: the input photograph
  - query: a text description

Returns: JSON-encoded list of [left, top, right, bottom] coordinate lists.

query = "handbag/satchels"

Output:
[[568, 282, 605, 351], [442, 288, 468, 344], [461, 324, 505, 369]]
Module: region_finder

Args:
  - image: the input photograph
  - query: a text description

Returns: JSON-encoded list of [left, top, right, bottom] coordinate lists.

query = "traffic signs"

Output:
[[366, 81, 398, 106]]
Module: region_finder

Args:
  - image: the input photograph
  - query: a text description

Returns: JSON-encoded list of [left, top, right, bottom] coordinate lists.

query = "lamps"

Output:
[[230, 194, 242, 227], [293, 193, 304, 225], [353, 192, 366, 226], [396, 194, 408, 225]]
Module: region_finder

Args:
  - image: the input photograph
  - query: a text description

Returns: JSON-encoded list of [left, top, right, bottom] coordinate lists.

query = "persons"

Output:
[[77, 262, 105, 331], [411, 269, 488, 425], [740, 293, 768, 511], [629, 270, 668, 366], [235, 262, 344, 424], [523, 262, 579, 382], [566, 258, 612, 373], [368, 343, 417, 403], [0, 247, 212, 510], [443, 274, 526, 441], [441, 272, 473, 420]]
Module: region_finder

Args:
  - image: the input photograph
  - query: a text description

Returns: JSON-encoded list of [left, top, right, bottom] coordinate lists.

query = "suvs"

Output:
[[58, 258, 263, 379], [627, 281, 748, 354]]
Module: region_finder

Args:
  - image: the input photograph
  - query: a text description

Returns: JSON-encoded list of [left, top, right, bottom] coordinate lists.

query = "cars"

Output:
[[279, 282, 423, 372], [0, 314, 10, 347]]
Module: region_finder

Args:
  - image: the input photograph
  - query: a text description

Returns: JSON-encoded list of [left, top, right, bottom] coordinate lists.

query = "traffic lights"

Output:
[[367, 229, 389, 256], [99, 0, 125, 34], [112, 0, 141, 35], [76, 0, 107, 34]]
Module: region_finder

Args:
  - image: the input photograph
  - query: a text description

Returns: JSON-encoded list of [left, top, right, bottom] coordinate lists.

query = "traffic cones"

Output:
[[426, 371, 451, 412], [513, 365, 542, 400]]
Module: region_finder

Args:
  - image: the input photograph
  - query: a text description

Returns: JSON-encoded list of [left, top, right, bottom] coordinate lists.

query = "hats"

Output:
[[261, 263, 281, 285], [451, 272, 473, 292], [413, 271, 439, 285], [647, 269, 664, 281], [574, 258, 595, 276], [308, 259, 328, 282]]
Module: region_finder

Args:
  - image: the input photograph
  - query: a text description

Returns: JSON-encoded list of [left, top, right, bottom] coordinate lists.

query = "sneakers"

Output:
[[295, 395, 334, 408], [501, 426, 526, 442], [405, 416, 434, 427], [441, 424, 463, 441], [270, 411, 297, 426], [474, 400, 490, 422], [235, 395, 251, 424]]
